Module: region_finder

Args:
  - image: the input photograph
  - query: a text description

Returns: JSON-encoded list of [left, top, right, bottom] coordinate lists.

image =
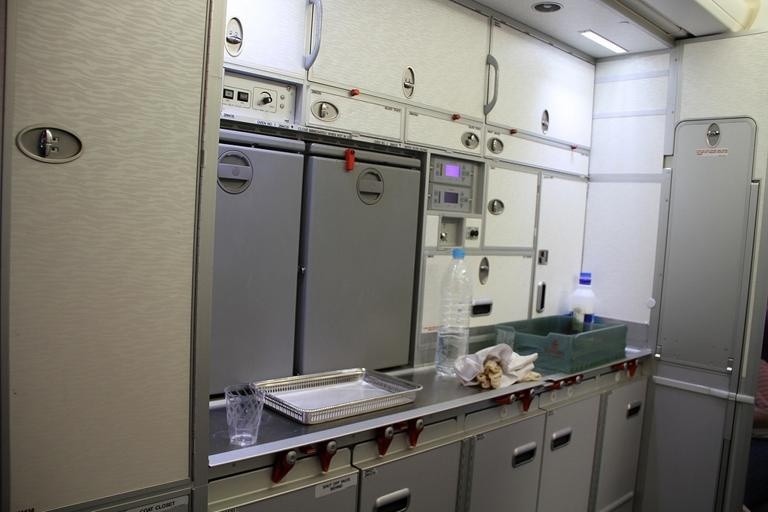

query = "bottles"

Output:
[[572, 272, 596, 332], [431, 248, 475, 377]]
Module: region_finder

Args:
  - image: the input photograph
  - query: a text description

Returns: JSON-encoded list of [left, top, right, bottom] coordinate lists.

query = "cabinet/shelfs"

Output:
[[530, 171, 588, 319], [420, 254, 535, 332], [0, 0, 226, 512], [225, 0, 598, 178], [479, 160, 540, 252], [594, 377, 646, 510], [208, 378, 602, 512]]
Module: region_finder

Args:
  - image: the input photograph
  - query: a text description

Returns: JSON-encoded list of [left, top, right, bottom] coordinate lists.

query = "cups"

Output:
[[224, 384, 266, 447]]
